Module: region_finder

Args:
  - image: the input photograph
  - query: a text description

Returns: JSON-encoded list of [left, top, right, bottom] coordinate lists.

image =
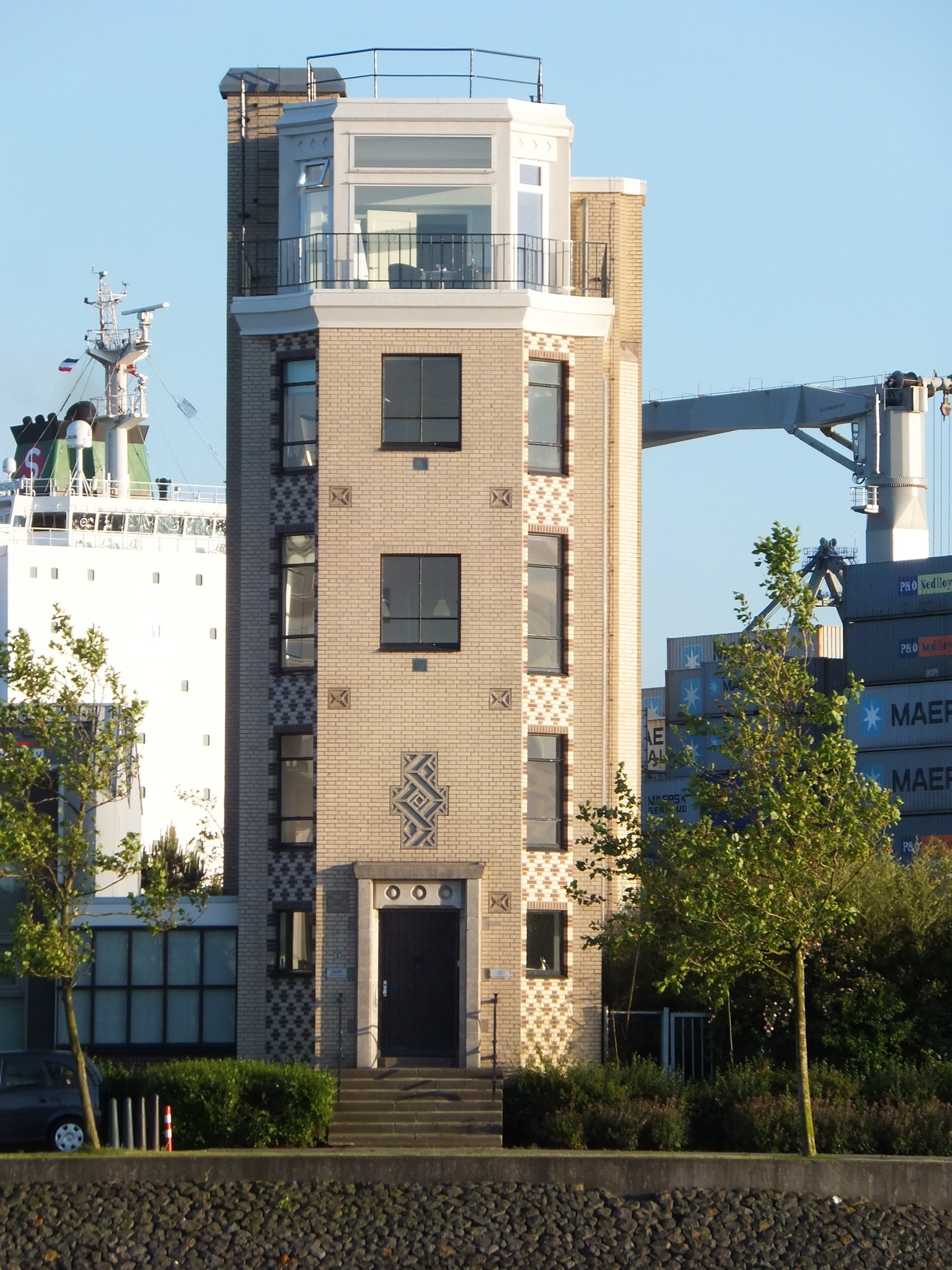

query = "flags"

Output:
[[127, 363, 137, 377], [59, 358, 80, 372]]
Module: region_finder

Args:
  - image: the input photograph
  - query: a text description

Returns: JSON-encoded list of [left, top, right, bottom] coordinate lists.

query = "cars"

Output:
[[0, 1049, 106, 1153]]
